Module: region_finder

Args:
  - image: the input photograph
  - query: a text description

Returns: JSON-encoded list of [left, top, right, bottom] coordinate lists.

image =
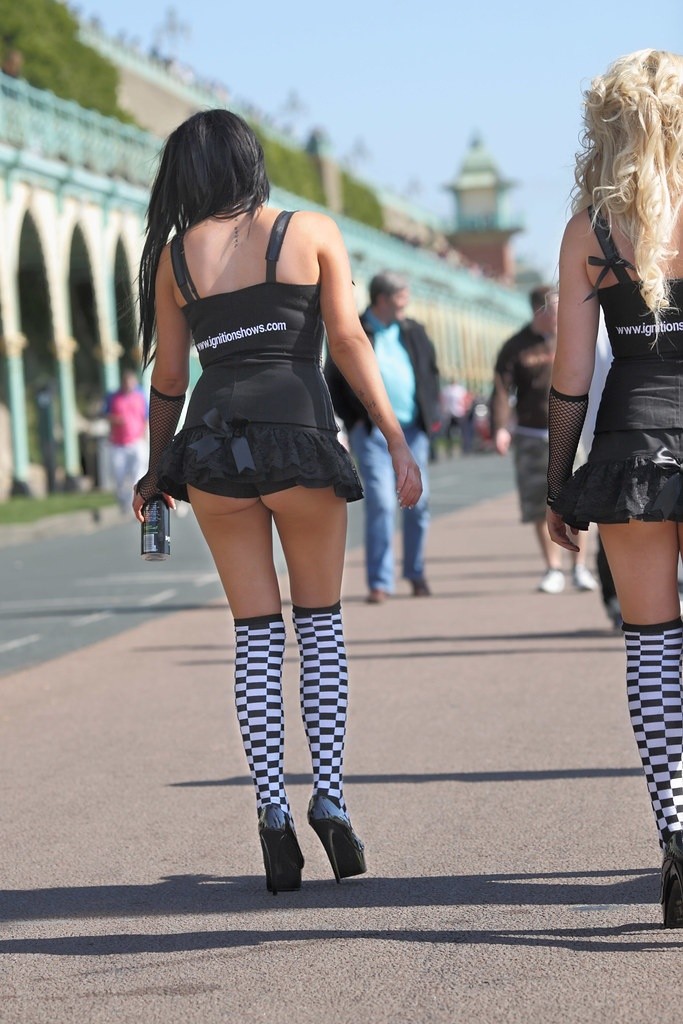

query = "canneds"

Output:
[[141, 495, 171, 560]]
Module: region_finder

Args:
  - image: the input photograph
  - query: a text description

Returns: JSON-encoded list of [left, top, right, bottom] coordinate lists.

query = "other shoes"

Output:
[[367, 591, 388, 602], [414, 578, 429, 596], [538, 570, 565, 593], [571, 567, 597, 590]]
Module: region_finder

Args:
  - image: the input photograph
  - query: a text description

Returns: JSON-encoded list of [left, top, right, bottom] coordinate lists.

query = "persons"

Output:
[[133, 112, 423, 895], [430, 371, 514, 462], [491, 287, 599, 594], [103, 369, 149, 513], [544, 49, 682, 930], [323, 271, 441, 604]]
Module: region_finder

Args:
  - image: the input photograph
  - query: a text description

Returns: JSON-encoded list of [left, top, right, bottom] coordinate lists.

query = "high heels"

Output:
[[660, 830, 683, 928], [258, 803, 304, 895], [307, 794, 367, 881]]
